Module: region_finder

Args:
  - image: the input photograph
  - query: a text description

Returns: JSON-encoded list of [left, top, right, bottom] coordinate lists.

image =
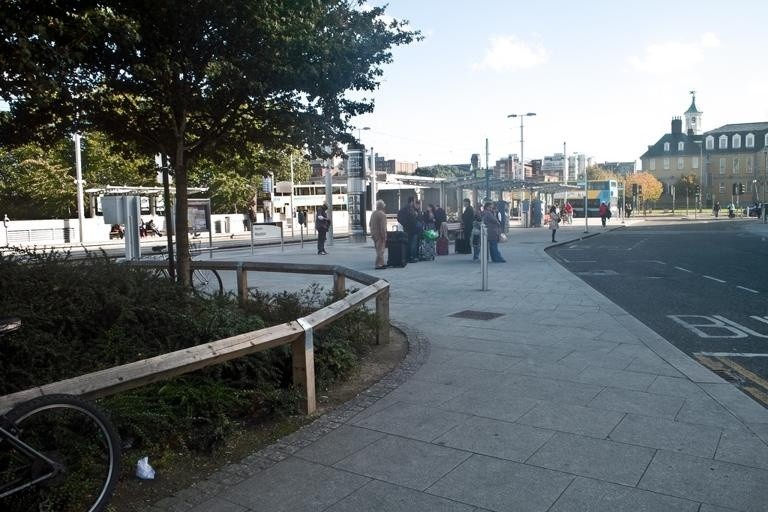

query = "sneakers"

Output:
[[320, 251, 328, 254]]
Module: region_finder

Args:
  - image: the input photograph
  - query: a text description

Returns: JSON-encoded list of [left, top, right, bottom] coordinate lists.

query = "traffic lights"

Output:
[[739, 183, 745, 195], [632, 184, 637, 197]]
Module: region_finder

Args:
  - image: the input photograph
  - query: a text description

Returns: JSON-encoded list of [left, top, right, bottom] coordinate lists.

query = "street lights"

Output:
[[507, 112, 536, 180], [693, 141, 703, 213], [358, 126, 371, 143]]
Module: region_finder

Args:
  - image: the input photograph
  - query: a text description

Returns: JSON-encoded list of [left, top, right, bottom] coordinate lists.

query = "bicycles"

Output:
[[151, 240, 223, 302], [0, 317, 121, 512]]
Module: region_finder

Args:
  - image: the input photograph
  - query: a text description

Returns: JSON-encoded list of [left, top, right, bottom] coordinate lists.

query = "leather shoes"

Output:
[[376, 264, 388, 269], [500, 259, 506, 262]]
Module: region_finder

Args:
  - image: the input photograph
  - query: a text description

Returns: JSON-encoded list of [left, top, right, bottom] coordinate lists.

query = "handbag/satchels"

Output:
[[607, 209, 611, 219], [498, 233, 508, 243]]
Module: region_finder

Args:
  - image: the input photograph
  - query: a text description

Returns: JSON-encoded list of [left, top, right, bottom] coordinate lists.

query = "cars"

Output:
[[743, 202, 768, 217]]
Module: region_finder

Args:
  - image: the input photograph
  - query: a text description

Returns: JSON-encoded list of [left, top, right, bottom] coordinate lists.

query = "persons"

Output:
[[606, 209, 612, 219], [727, 200, 735, 218], [755, 197, 763, 218], [264, 206, 270, 223], [461, 196, 509, 263], [626, 200, 632, 218], [712, 199, 721, 217], [314, 201, 331, 255], [292, 206, 308, 227], [147, 219, 162, 237], [617, 195, 624, 218], [599, 202, 608, 227], [564, 202, 574, 224], [549, 206, 559, 242], [369, 199, 389, 269], [140, 219, 147, 238], [111, 223, 123, 239], [397, 195, 447, 263]]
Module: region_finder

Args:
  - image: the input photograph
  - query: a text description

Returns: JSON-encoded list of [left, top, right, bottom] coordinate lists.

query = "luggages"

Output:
[[388, 225, 407, 267], [419, 225, 436, 261], [438, 230, 449, 256], [455, 229, 466, 253]]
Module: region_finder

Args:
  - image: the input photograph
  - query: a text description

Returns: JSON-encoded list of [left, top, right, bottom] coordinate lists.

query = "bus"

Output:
[[549, 179, 618, 218]]
